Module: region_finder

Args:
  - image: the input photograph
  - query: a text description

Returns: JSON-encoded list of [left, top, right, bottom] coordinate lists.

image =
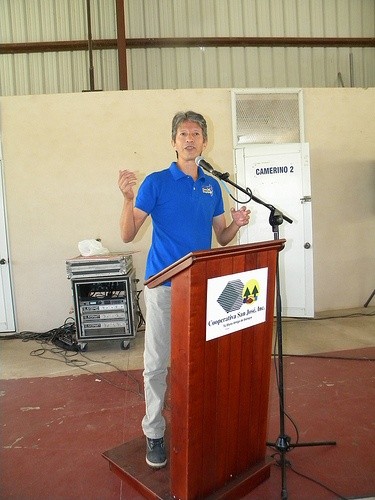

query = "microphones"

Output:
[[194, 156, 217, 176]]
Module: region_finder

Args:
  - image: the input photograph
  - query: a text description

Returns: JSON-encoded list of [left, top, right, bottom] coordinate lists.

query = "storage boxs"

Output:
[[65, 252, 134, 279]]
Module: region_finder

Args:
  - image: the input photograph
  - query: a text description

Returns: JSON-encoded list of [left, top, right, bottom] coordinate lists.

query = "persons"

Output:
[[119, 110, 250, 468]]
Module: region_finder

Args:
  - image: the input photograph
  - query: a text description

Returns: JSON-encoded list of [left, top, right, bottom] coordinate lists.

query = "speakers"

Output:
[[72, 270, 140, 351]]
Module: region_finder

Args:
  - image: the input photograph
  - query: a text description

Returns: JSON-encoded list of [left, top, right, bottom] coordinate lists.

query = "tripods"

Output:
[[262, 207, 340, 498]]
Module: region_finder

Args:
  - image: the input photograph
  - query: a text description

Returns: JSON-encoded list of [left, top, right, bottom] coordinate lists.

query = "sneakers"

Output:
[[145, 436, 168, 468]]
